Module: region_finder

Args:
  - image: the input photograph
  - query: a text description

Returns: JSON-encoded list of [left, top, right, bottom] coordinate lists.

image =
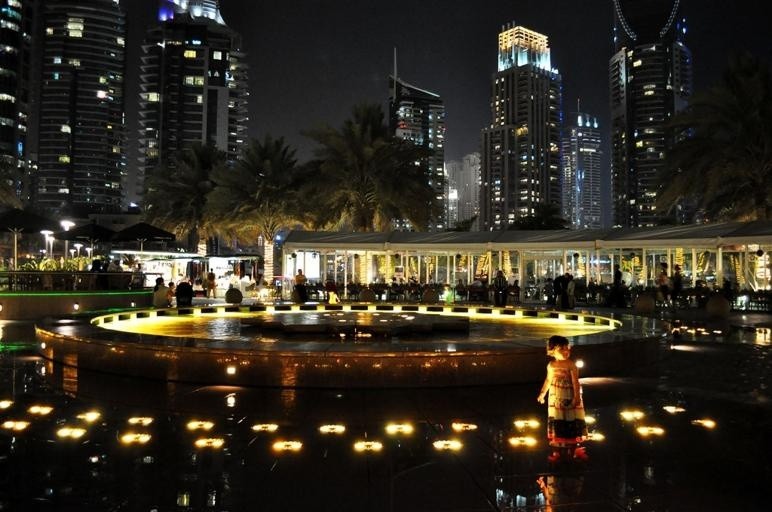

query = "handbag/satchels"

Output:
[[554, 419, 585, 438]]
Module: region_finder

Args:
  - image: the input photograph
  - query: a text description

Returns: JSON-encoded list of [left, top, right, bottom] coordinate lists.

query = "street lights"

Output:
[[40, 220, 91, 270]]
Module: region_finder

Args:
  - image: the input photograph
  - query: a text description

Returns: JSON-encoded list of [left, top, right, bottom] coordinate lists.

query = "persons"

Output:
[[613, 264, 622, 304], [544, 273, 577, 309], [151, 276, 172, 307], [206, 268, 217, 298], [536, 335, 590, 463], [455, 279, 468, 300], [90, 258, 146, 290], [493, 271, 508, 305], [168, 282, 176, 306], [295, 269, 308, 301], [671, 263, 683, 306], [655, 263, 671, 308], [622, 267, 631, 283], [391, 274, 434, 301]]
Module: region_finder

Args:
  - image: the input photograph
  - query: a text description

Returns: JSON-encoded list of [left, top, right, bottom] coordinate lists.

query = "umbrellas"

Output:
[[0, 208, 61, 270], [48, 222, 118, 265], [112, 223, 176, 258]]
